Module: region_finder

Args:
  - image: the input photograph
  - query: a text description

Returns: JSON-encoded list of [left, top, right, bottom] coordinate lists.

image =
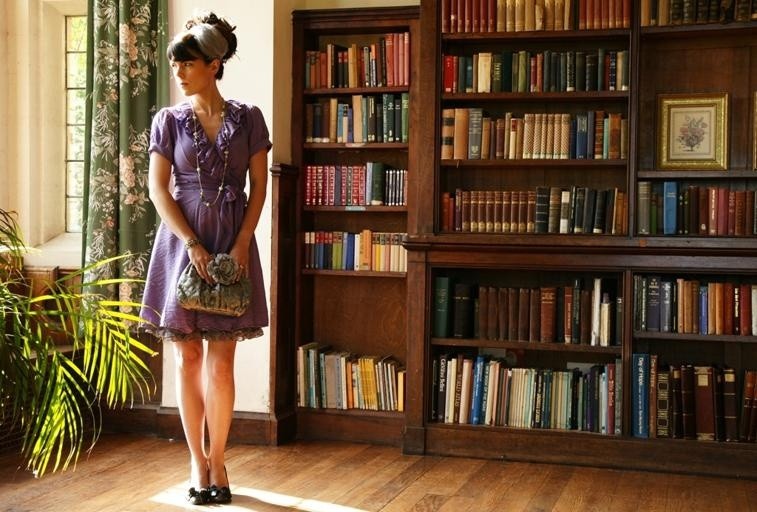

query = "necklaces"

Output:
[[188, 96, 231, 208]]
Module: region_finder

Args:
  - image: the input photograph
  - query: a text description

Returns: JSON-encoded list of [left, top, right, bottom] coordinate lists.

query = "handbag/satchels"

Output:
[[176, 254, 250, 316]]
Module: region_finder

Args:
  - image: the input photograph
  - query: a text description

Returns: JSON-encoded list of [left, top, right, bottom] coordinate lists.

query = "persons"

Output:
[[135, 9, 274, 506]]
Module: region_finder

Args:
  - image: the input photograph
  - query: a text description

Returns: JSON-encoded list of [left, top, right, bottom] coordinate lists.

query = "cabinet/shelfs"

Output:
[[268, 0, 757, 482]]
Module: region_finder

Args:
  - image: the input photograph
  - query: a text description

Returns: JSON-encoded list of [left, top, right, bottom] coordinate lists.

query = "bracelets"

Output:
[[184, 238, 203, 250]]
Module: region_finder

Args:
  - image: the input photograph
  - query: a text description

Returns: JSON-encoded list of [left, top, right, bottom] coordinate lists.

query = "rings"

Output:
[[239, 264, 244, 269]]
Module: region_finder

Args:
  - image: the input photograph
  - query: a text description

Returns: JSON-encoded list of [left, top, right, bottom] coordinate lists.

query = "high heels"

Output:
[[188, 465, 232, 505]]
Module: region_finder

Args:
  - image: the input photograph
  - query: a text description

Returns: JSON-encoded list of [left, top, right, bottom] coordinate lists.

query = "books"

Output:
[[428, 186, 627, 435], [440, 0, 757, 33], [304, 229, 408, 273], [304, 162, 408, 207], [440, 48, 629, 161], [305, 32, 410, 143], [633, 181, 757, 442], [297, 342, 406, 412]]
